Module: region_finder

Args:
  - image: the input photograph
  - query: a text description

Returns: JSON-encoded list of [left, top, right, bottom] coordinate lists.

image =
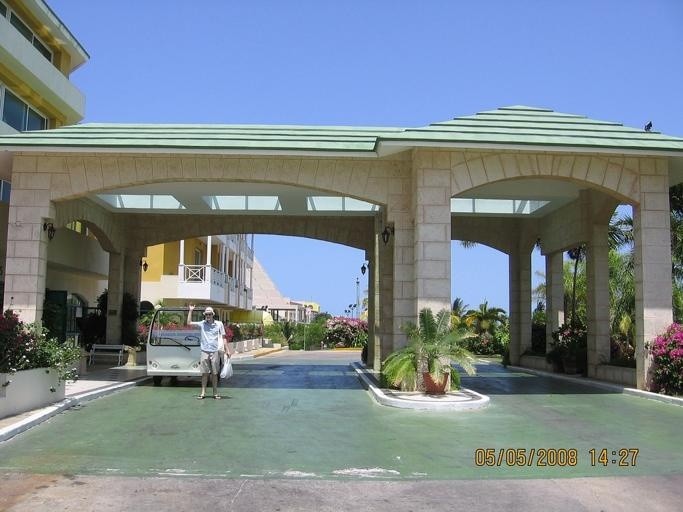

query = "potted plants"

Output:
[[381, 308, 481, 397], [546, 321, 587, 375]]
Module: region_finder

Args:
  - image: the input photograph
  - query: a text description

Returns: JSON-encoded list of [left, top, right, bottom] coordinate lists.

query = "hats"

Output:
[[202, 307, 215, 316]]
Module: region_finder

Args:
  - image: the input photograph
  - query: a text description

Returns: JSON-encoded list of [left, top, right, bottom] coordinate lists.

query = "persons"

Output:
[[186, 304, 232, 399]]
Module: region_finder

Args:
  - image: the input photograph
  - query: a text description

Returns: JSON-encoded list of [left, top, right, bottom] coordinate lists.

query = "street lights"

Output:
[[361, 262, 368, 275], [344, 277, 360, 317]]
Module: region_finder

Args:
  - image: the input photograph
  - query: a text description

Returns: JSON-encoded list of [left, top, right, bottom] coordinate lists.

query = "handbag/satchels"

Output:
[[216, 320, 224, 355]]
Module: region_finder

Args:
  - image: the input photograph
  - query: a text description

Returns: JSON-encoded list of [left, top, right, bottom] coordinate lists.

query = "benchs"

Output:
[[89, 344, 125, 366], [159, 336, 201, 345]]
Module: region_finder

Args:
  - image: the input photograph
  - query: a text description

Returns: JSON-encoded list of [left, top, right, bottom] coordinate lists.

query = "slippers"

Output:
[[196, 394, 221, 400]]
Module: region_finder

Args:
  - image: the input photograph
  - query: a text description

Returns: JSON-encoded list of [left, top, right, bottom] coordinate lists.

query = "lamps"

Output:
[[140, 259, 148, 272], [43, 222, 55, 240], [381, 225, 394, 245], [361, 263, 371, 275]]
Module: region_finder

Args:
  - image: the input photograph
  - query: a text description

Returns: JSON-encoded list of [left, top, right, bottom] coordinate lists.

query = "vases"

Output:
[[0, 366, 65, 420]]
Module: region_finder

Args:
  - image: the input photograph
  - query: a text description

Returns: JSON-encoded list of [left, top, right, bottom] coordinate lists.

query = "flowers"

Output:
[[644, 322, 683, 397], [0, 309, 83, 394]]
